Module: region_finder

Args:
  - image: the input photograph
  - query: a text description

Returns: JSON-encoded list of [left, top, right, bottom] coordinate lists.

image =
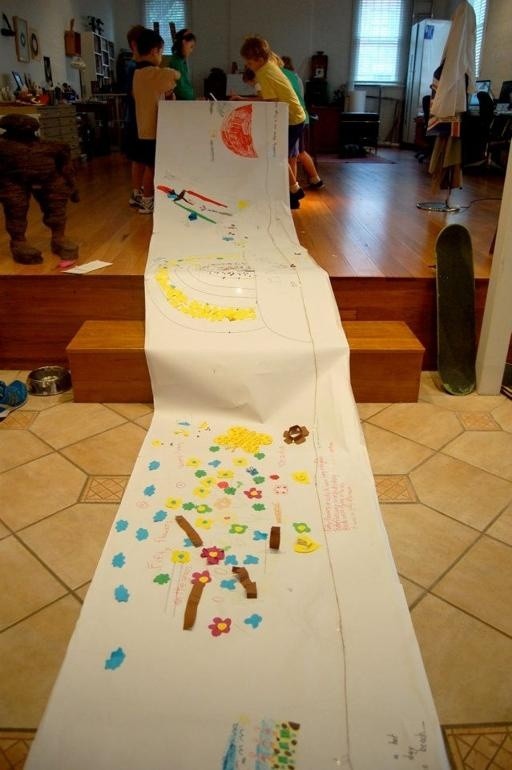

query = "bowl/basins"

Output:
[[25, 366, 72, 397]]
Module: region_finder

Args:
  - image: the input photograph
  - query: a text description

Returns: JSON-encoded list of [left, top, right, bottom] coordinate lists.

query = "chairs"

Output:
[[410, 90, 512, 176]]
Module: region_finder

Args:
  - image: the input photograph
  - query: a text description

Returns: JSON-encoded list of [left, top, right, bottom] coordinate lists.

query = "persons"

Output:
[[170, 29, 195, 99], [279, 65, 324, 189], [0, 378, 30, 418], [1, 113, 82, 265], [229, 37, 305, 209], [118, 26, 148, 165], [242, 67, 262, 97], [127, 29, 182, 215], [280, 56, 304, 97]]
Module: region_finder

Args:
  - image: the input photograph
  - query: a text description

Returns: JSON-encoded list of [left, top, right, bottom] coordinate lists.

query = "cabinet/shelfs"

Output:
[[73, 102, 115, 161], [309, 103, 345, 150], [1, 105, 82, 164], [79, 32, 115, 97]]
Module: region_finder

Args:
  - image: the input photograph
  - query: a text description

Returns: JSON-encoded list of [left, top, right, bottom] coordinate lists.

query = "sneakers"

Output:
[[290, 196, 300, 209], [290, 186, 305, 200], [127, 188, 144, 208], [307, 179, 327, 192], [0, 380, 29, 422], [138, 196, 153, 215]]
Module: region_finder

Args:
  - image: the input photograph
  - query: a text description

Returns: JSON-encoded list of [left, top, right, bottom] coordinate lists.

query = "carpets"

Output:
[[314, 151, 400, 165]]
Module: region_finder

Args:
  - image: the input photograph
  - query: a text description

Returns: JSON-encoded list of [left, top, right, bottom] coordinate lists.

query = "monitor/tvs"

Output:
[[469, 79, 492, 106]]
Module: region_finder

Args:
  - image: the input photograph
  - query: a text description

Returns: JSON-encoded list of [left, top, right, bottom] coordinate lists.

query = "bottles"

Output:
[[310, 50, 328, 81]]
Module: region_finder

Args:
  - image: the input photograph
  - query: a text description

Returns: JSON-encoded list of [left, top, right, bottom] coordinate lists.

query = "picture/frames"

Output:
[[12, 15, 32, 64], [12, 71, 25, 92]]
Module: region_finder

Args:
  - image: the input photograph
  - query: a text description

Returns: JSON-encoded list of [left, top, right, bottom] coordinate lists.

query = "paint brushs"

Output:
[[24, 72, 38, 89]]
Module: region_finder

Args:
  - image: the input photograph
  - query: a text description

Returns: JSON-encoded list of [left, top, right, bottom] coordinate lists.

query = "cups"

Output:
[[347, 90, 366, 113]]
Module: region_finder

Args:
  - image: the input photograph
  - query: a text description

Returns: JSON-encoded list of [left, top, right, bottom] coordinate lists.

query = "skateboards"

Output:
[[435, 223, 475, 398]]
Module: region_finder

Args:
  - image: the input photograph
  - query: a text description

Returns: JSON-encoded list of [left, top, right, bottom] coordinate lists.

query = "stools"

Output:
[[338, 110, 382, 151]]
